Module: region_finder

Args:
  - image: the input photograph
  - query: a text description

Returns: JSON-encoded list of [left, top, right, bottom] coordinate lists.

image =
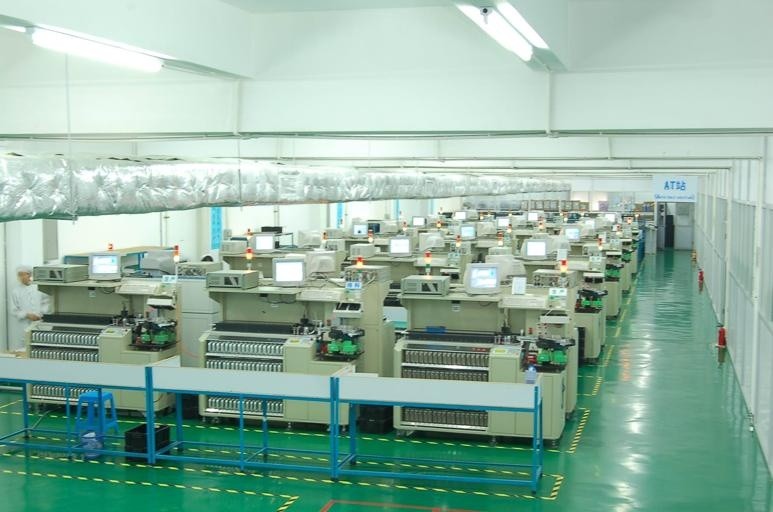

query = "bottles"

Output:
[[524, 364, 537, 384]]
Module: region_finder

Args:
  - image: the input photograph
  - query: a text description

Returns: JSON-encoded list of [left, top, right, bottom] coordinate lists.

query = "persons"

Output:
[[8, 263, 49, 350]]
